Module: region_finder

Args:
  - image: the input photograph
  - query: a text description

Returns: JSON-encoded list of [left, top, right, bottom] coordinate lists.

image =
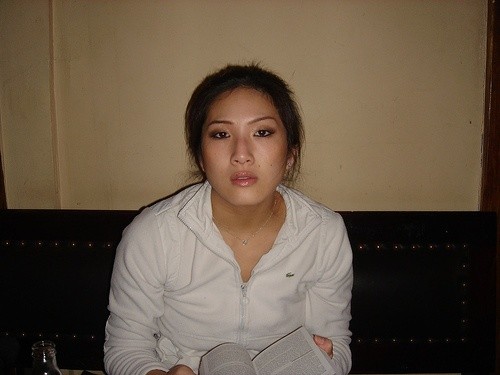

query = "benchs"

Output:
[[0, 208, 500, 375]]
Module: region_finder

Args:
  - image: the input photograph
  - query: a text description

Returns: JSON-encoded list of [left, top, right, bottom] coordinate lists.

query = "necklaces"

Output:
[[212, 196, 277, 247]]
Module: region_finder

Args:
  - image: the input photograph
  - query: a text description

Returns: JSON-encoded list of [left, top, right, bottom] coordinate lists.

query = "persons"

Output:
[[103, 59, 352, 375]]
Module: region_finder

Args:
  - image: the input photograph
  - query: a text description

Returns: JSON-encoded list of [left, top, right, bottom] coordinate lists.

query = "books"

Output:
[[197, 326, 336, 374]]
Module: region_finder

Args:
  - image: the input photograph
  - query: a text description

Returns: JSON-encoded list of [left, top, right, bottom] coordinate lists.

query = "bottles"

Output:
[[30, 341, 63, 375]]
